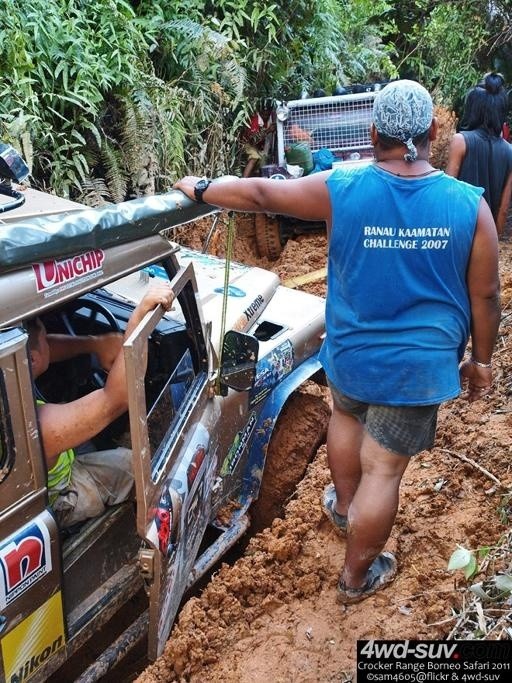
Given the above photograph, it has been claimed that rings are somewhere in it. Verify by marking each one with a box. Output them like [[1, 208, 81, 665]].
[[479, 387, 486, 391]]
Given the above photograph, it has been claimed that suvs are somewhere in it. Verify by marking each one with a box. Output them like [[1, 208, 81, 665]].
[[255, 82, 391, 260], [1, 144, 327, 682]]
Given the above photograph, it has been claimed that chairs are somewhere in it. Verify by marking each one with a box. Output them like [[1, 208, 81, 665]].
[[56, 499, 138, 616]]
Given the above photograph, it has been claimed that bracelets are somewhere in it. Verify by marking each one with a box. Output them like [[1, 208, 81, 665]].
[[470, 355, 493, 373]]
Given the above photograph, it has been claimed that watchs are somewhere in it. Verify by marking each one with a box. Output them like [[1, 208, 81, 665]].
[[192, 175, 214, 205]]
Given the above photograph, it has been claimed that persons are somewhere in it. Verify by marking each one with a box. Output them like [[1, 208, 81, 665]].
[[169, 77, 506, 614], [445, 70, 512, 239], [21, 279, 179, 533], [234, 79, 386, 178]]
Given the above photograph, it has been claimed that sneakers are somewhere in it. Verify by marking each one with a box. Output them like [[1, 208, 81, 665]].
[[337, 552, 398, 604], [321, 484, 348, 538]]
